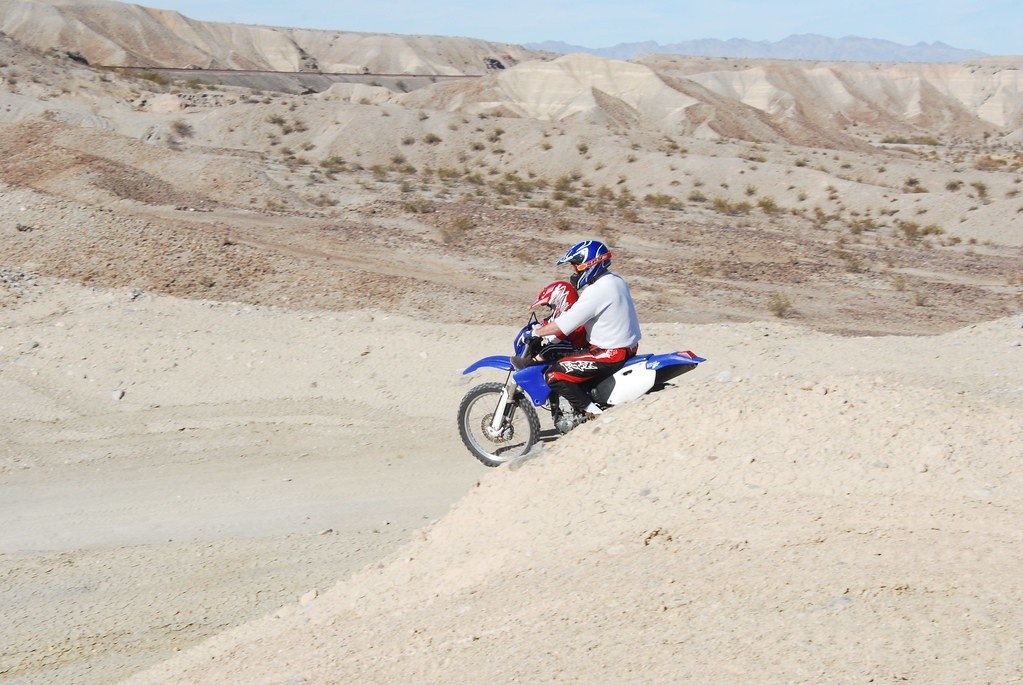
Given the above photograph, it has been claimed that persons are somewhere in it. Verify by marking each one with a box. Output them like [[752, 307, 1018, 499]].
[[510, 240, 643, 434]]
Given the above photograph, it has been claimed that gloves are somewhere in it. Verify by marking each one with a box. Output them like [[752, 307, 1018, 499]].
[[516, 329, 533, 347]]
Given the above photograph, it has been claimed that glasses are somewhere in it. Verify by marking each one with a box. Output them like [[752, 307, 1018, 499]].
[[571, 262, 580, 275]]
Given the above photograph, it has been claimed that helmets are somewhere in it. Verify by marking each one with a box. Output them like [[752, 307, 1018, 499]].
[[555, 240, 613, 291], [528, 280, 580, 328]]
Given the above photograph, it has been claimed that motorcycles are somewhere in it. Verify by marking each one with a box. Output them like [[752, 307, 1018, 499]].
[[457, 312, 706, 467]]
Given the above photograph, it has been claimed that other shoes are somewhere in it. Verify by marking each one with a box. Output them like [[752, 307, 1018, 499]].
[[509, 356, 543, 370]]
